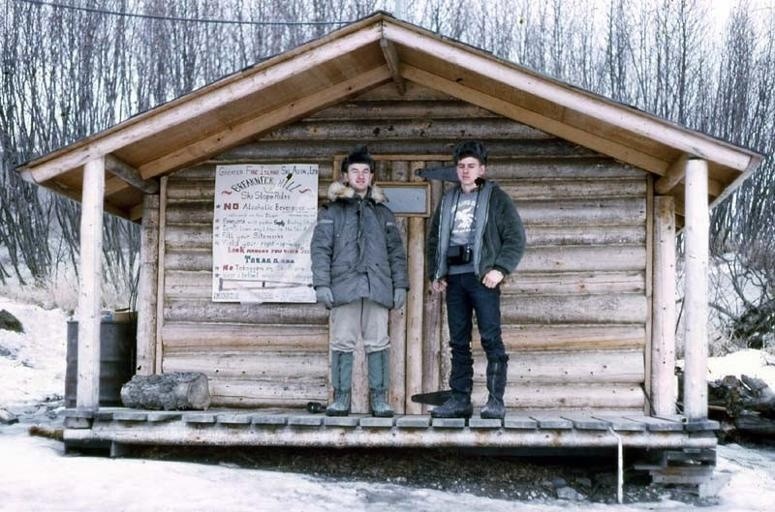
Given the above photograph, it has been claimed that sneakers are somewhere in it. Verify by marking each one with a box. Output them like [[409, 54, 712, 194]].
[[326, 390, 351, 416], [369, 390, 394, 417]]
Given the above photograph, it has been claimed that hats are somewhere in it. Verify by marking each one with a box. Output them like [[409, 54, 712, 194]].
[[453, 139, 488, 161], [341, 152, 376, 173]]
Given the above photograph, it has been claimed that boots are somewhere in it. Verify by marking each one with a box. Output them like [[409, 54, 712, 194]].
[[429, 357, 476, 419], [478, 360, 509, 418]]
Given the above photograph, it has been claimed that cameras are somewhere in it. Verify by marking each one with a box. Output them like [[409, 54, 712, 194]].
[[445, 246, 473, 266]]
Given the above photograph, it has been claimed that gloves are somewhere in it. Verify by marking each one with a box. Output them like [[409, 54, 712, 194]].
[[394, 287, 406, 310], [316, 286, 334, 309]]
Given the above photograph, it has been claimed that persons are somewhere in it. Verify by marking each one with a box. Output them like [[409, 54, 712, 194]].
[[310, 150, 409, 416], [432, 148, 526, 418]]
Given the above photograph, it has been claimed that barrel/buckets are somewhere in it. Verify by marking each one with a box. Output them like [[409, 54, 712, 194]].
[[65, 320, 134, 410]]
